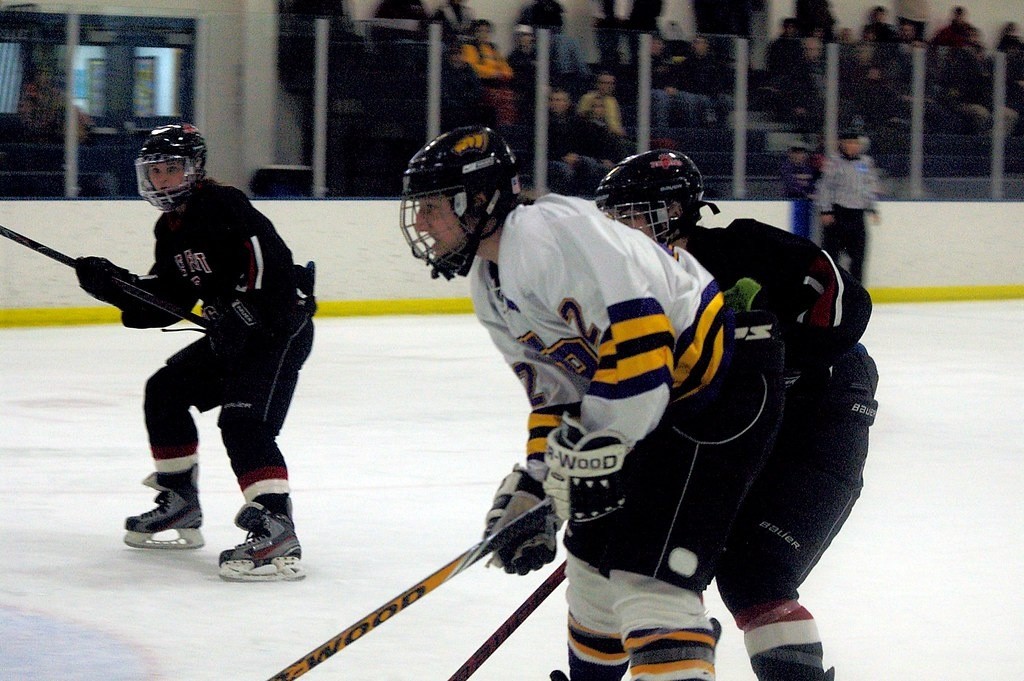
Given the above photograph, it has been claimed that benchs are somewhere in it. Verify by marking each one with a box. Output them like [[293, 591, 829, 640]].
[[503, 63, 1024, 202]]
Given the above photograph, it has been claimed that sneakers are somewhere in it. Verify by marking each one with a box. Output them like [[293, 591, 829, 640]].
[[217, 497, 307, 583], [812, 667, 835, 681], [710, 618, 721, 657], [549, 670, 570, 681], [123, 462, 206, 549]]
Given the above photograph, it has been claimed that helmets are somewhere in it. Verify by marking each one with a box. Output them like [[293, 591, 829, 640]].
[[595, 148, 705, 247], [133, 123, 208, 216], [400, 125, 520, 282]]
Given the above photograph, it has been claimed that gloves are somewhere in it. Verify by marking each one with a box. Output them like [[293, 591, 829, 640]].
[[76, 256, 139, 311], [542, 411, 636, 537], [481, 463, 564, 575], [719, 274, 780, 349], [205, 298, 274, 359]]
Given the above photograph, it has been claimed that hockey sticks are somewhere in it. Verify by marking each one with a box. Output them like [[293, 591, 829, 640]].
[[262, 500, 568, 681], [0, 225, 214, 329]]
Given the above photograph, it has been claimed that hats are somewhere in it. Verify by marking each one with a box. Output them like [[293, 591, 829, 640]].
[[510, 24, 534, 36]]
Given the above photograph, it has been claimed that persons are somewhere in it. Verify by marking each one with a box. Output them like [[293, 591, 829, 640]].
[[449, 4, 1023, 200], [74, 122, 319, 582], [398, 125, 785, 680], [815, 129, 880, 285], [592, 147, 881, 681]]
